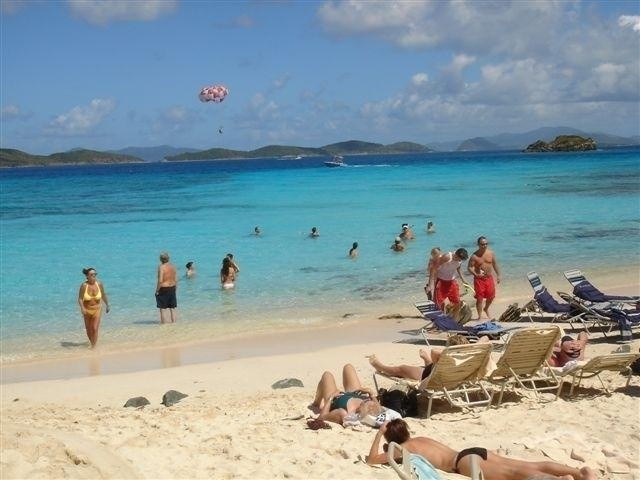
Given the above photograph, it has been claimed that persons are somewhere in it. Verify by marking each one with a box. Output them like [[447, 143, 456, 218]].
[[220, 258, 236, 290], [225, 253, 241, 273], [367, 418, 599, 480], [425, 221, 436, 232], [466, 235, 501, 320], [154, 252, 179, 325], [424, 248, 470, 309], [254, 224, 261, 235], [427, 247, 442, 269], [184, 260, 195, 278], [310, 363, 381, 424], [77, 266, 111, 349], [390, 237, 404, 252], [349, 240, 359, 257], [310, 225, 318, 236], [370, 334, 489, 381], [546, 329, 588, 367], [400, 222, 415, 240]]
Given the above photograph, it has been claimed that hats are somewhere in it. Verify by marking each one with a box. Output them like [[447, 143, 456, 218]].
[[562, 336, 580, 358]]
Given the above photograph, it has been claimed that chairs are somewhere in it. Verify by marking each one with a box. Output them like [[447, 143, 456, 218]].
[[539, 352, 636, 404], [373, 342, 493, 420], [387, 441, 483, 480], [412, 270, 640, 344], [472, 327, 561, 410]]
